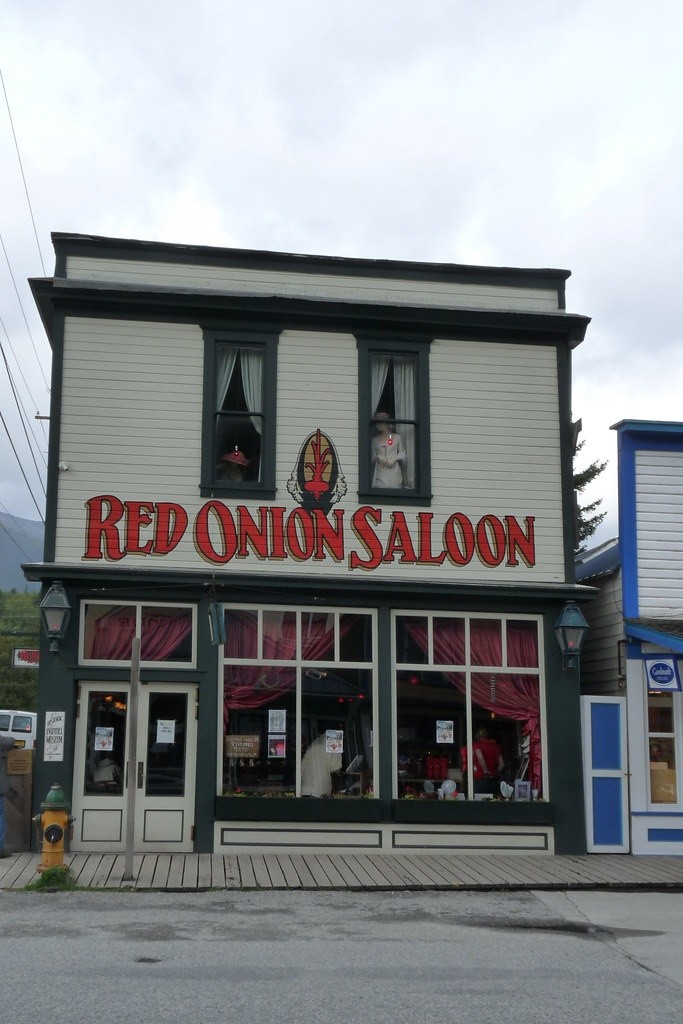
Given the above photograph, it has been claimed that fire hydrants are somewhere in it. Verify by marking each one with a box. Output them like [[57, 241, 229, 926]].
[[32, 784, 76, 870]]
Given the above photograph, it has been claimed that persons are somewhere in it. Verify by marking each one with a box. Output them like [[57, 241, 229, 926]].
[[216, 446, 248, 481], [303, 732, 342, 798], [474, 727, 504, 793], [90, 751, 122, 784], [0, 735, 14, 858], [370, 413, 406, 488], [460, 735, 467, 794]]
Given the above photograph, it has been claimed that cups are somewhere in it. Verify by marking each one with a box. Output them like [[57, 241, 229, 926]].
[[532, 789, 538, 801], [456, 793, 464, 800], [437, 789, 444, 800]]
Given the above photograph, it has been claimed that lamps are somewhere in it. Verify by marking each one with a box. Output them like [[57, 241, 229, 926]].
[[36, 580, 71, 652], [553, 599, 588, 669]]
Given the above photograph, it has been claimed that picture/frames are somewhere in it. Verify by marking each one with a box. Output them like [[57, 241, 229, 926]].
[[514, 781, 530, 801]]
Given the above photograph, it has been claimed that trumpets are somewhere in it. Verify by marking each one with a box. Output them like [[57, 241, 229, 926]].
[[306, 667, 327, 680]]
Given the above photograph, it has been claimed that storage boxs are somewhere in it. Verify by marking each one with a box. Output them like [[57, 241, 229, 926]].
[[649, 762, 675, 803]]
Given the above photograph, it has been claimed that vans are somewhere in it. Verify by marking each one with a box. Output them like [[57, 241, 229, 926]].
[[0, 709, 37, 750]]
